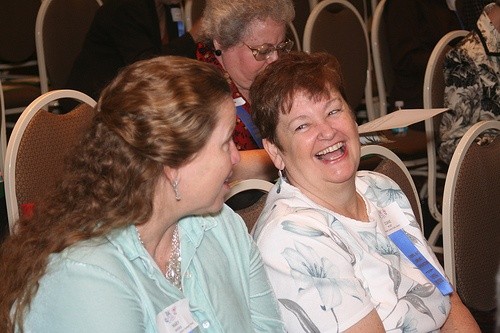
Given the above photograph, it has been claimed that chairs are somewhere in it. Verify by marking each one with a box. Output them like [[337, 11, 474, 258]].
[[0, 0, 500, 333]]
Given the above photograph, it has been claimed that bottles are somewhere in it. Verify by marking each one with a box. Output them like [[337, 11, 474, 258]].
[[392, 100, 408, 136]]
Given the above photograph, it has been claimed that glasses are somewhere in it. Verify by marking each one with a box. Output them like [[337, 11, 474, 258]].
[[234, 36, 294, 61]]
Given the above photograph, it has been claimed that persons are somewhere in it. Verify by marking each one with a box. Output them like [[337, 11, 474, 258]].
[[0, 56, 287, 333], [440, 0, 500, 164], [59, 0, 208, 114], [197, 0, 294, 209], [249, 53, 482, 333]]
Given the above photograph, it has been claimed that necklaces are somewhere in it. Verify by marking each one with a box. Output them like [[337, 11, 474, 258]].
[[136, 224, 181, 290]]
